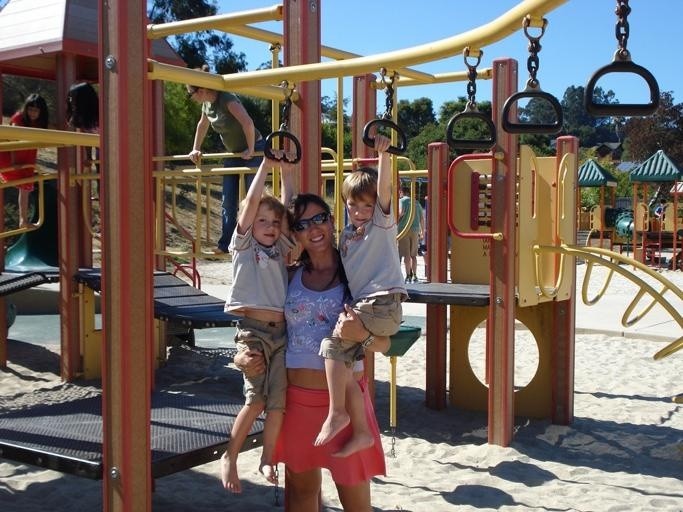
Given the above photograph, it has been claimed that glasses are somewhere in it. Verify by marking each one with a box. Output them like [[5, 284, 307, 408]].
[[187, 90, 198, 97], [293, 212, 331, 232]]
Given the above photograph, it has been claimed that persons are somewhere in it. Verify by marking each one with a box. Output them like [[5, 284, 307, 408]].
[[217, 149, 298, 492], [62, 81, 99, 138], [231, 192, 393, 511], [654, 198, 666, 221], [311, 131, 408, 459], [182, 64, 266, 258], [0, 91, 49, 230], [396, 183, 425, 284]]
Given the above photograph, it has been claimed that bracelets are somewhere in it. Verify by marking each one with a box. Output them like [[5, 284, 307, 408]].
[[360, 334, 375, 351]]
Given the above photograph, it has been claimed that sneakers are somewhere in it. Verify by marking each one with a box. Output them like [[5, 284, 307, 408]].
[[406, 274, 418, 284]]
